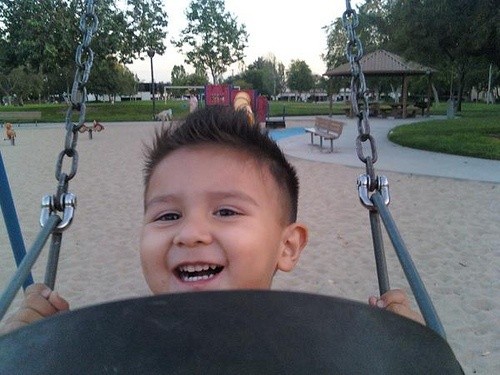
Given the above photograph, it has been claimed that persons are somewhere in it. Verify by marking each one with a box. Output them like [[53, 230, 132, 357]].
[[0, 104, 428, 341], [188, 89, 198, 114]]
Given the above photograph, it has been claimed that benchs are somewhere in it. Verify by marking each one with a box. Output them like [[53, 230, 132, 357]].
[[304, 116, 344, 154], [379, 107, 393, 118], [0, 111, 41, 128], [394, 109, 417, 119]]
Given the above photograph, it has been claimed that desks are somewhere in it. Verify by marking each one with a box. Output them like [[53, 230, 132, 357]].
[[389, 102, 410, 109]]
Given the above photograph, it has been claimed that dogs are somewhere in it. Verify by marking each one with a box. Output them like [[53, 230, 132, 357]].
[[155, 108, 173, 121], [5, 122, 16, 139]]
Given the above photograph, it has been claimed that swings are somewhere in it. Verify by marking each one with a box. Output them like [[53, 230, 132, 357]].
[[0, 2, 466, 375]]
